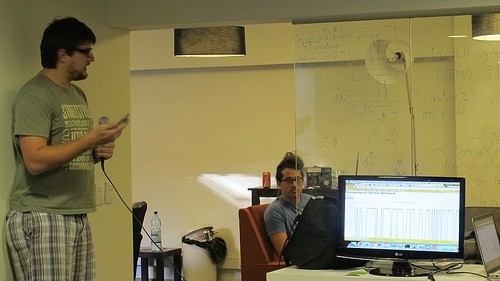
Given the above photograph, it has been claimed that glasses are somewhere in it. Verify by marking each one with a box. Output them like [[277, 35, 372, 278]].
[[60, 46, 92, 56], [278, 177, 303, 184]]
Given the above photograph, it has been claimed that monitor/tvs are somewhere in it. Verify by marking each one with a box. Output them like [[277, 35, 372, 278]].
[[336, 174, 466, 276]]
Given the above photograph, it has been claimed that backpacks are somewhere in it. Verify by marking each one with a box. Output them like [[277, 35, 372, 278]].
[[278, 195, 370, 270]]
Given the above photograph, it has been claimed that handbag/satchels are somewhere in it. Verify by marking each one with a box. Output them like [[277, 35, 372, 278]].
[[210, 237, 227, 264]]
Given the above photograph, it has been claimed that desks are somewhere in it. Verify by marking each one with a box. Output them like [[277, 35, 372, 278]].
[[266, 258, 500, 281], [248, 185, 338, 205], [138, 247, 182, 281]]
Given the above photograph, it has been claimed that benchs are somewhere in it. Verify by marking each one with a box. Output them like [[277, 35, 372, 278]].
[[239, 203, 288, 281]]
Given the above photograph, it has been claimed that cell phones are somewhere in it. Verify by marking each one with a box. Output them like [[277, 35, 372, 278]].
[[116, 113, 129, 123]]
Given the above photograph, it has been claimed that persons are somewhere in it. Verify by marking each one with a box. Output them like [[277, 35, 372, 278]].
[[262, 152, 315, 264], [3, 15, 130, 281]]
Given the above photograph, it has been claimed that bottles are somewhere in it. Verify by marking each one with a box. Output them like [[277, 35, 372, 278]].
[[150, 210, 162, 252]]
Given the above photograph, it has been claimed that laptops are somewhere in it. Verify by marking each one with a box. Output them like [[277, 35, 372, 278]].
[[471, 212, 500, 279]]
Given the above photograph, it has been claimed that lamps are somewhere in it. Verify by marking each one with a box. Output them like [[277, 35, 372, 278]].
[[472, 13, 500, 40], [174, 25, 247, 57]]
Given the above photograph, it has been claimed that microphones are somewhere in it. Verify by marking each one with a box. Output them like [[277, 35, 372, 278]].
[[99, 116, 110, 169]]
